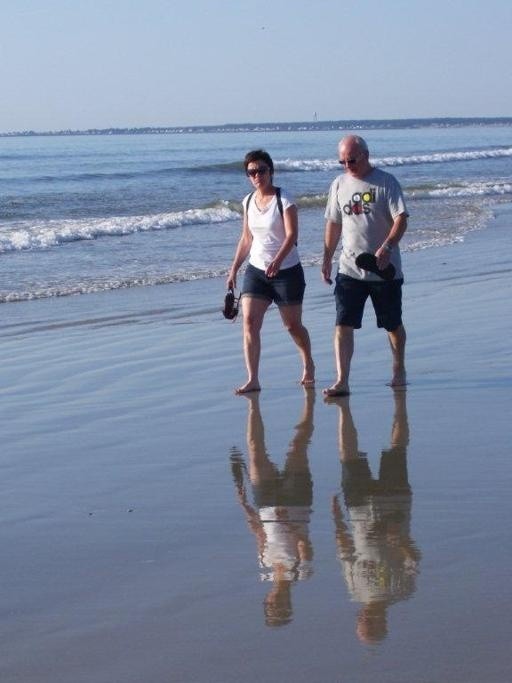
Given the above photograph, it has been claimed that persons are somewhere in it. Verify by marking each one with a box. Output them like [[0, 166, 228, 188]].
[[225, 151, 316, 394], [324, 392, 421, 646], [322, 135, 406, 397], [230, 384, 316, 628]]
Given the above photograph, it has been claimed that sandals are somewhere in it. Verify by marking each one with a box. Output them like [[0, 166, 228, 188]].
[[224, 289, 241, 322]]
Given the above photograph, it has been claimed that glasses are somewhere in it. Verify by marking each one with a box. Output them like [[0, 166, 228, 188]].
[[246, 167, 269, 177]]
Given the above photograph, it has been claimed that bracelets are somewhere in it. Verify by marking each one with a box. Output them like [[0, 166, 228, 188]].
[[381, 244, 395, 254]]
